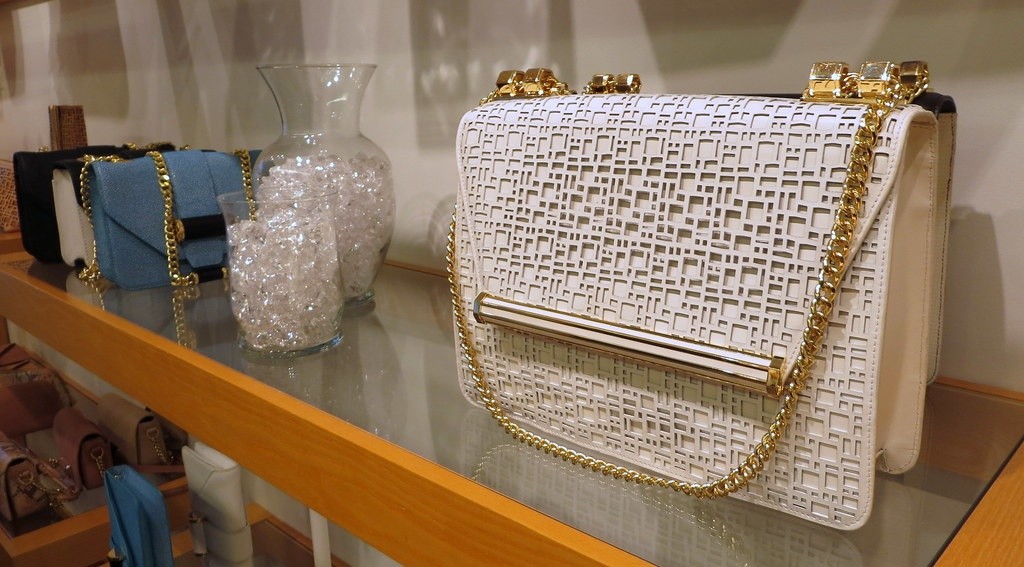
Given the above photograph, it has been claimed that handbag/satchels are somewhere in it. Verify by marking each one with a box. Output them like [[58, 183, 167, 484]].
[[45, 141, 177, 269], [0, 343, 29, 366], [12, 142, 137, 264], [0, 145, 49, 234], [446, 60, 958, 531], [0, 358, 46, 385], [0, 430, 80, 523], [52, 406, 115, 492], [96, 393, 169, 469], [0, 368, 74, 438], [87, 145, 278, 292]]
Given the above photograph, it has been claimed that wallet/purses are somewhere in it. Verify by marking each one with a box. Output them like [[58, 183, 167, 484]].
[[102, 464, 175, 567], [182, 440, 255, 567], [48, 104, 88, 150]]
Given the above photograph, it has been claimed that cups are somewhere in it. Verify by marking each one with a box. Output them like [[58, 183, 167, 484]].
[[216, 188, 344, 357]]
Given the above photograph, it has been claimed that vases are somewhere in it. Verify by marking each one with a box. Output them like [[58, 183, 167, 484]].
[[217, 186, 345, 359], [254, 65, 396, 314]]
